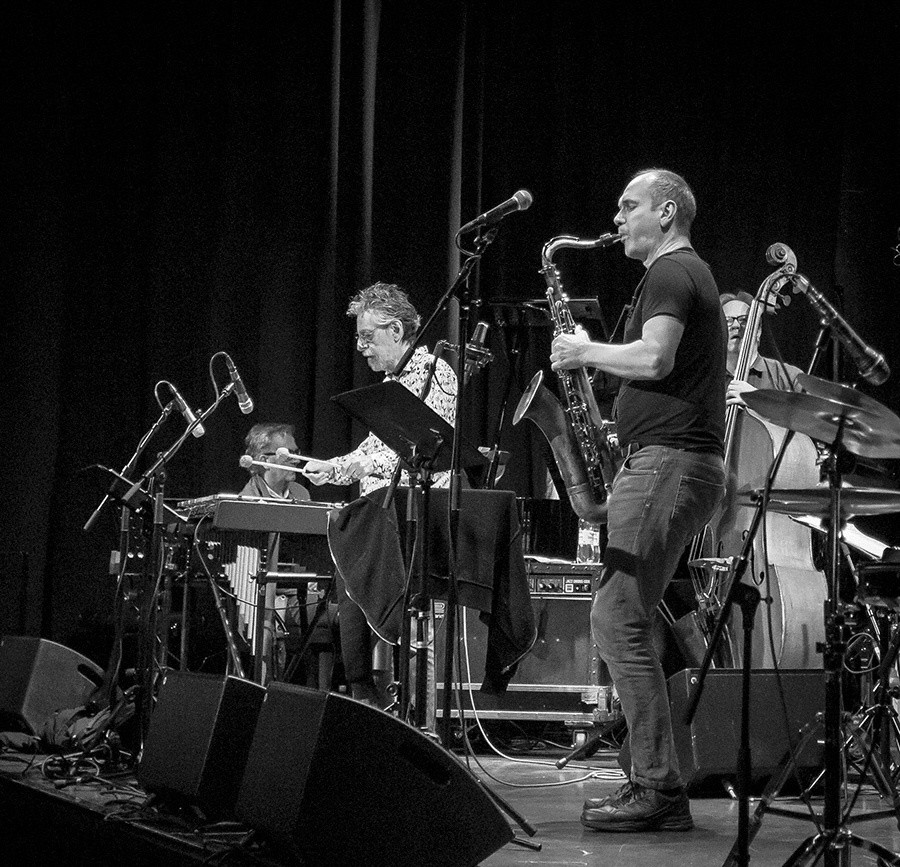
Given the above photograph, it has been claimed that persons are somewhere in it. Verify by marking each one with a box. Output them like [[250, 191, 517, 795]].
[[548, 166, 726, 834], [300, 283, 460, 710], [715, 290, 818, 407], [234, 422, 344, 645]]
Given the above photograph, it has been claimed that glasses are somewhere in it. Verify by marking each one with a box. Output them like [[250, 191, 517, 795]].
[[352, 325, 382, 341], [725, 314, 749, 327], [262, 447, 303, 460]]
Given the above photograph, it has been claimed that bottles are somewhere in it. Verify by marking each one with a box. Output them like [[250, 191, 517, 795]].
[[577, 518, 600, 564]]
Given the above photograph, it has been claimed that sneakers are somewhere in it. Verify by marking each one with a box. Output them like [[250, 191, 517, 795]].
[[579, 782, 694, 831], [585, 782, 635, 812]]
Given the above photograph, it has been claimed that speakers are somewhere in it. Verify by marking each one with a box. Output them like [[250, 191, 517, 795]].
[[0, 636, 105, 737], [614, 668, 825, 795], [136, 669, 267, 815], [230, 680, 515, 867]]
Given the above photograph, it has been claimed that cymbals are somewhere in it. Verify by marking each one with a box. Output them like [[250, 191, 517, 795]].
[[739, 478, 900, 514], [797, 372, 899, 429], [739, 388, 900, 457]]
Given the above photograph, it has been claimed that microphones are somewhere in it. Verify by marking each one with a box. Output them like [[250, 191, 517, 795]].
[[463, 321, 489, 389], [170, 384, 207, 438], [459, 189, 533, 239], [224, 355, 255, 414], [790, 276, 891, 387]]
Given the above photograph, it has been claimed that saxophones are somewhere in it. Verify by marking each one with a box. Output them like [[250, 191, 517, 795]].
[[511, 228, 622, 520]]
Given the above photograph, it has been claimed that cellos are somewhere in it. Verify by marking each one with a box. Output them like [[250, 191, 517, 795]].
[[672, 241, 830, 670]]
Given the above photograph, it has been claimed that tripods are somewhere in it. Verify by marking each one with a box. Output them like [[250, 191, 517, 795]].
[[35, 398, 179, 770], [782, 319, 898, 867], [55, 383, 236, 789], [390, 222, 506, 759], [283, 340, 465, 722]]
